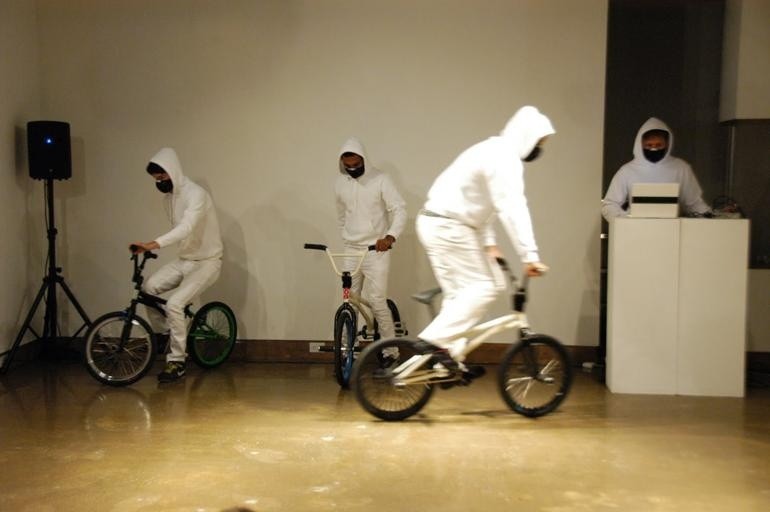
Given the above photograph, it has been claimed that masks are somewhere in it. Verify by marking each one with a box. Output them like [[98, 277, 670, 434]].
[[644, 149, 665, 163], [345, 165, 364, 178], [525, 147, 539, 161], [156, 179, 172, 193]]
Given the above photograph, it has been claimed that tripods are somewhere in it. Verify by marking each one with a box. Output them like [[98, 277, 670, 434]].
[[1, 180, 117, 379]]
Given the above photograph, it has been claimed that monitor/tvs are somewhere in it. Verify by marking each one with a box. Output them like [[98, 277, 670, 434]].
[[630, 183, 680, 217]]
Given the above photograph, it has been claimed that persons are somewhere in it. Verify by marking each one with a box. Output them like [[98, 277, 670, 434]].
[[127, 145, 224, 385], [598, 116, 715, 223], [415, 103, 557, 392], [331, 137, 407, 380]]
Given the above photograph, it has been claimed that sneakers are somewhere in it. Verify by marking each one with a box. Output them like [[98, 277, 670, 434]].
[[342, 347, 361, 368], [157, 335, 170, 354], [158, 361, 186, 383], [379, 357, 396, 368], [437, 366, 486, 389], [413, 341, 458, 372]]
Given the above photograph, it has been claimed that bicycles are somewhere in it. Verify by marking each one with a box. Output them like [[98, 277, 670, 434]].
[[345, 254, 570, 421], [301, 241, 402, 373], [75, 241, 241, 385]]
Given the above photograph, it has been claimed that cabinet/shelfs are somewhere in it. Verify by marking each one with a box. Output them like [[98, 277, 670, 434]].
[[604, 216, 751, 397], [719, 0, 769, 125]]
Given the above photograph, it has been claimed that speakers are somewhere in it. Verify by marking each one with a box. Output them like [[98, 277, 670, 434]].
[[27, 120, 73, 180]]
